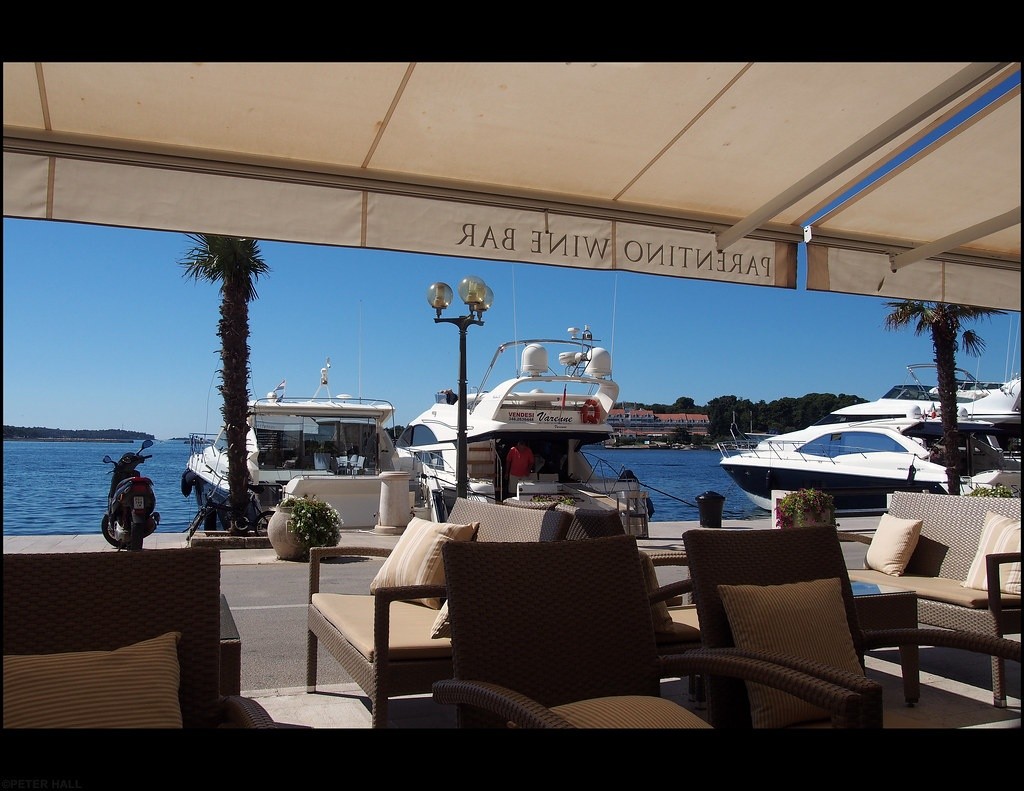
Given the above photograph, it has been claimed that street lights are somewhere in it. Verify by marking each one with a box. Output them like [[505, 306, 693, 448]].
[[428, 275, 495, 499]]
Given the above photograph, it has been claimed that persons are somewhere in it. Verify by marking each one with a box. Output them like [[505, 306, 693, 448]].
[[441, 389, 458, 405], [505, 440, 535, 497]]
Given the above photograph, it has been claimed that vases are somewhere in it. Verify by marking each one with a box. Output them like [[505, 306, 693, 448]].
[[267, 504, 312, 560], [793, 507, 831, 528]]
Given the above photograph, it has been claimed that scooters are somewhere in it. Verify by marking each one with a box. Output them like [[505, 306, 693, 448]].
[[101, 438, 161, 551]]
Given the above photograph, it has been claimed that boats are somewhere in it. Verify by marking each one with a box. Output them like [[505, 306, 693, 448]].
[[182, 356, 406, 535], [672, 442, 686, 450], [804, 362, 1022, 436], [394, 323, 655, 523], [710, 443, 739, 452], [715, 421, 1022, 519]]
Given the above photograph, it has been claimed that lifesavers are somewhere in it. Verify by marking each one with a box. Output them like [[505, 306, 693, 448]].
[[581, 398, 600, 424]]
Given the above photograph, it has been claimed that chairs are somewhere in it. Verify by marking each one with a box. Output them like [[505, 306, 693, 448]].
[[304, 491, 1023, 731], [336, 455, 365, 475], [0, 545, 277, 729], [282, 456, 298, 468]]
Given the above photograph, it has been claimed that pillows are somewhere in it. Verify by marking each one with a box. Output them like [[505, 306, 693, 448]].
[[506, 693, 714, 730], [716, 578, 866, 728], [370, 516, 480, 609], [861, 513, 923, 577], [2, 631, 183, 729], [961, 509, 1022, 596]]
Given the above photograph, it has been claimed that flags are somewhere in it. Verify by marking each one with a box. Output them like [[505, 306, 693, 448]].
[[273, 380, 285, 392], [932, 405, 936, 419]]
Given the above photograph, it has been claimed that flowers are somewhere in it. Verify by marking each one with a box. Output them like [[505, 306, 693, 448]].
[[773, 487, 841, 531], [276, 494, 344, 562], [964, 485, 1018, 499]]
